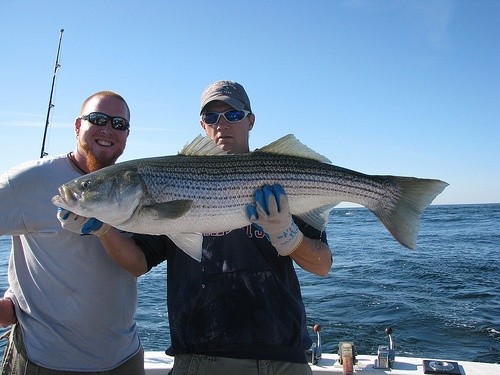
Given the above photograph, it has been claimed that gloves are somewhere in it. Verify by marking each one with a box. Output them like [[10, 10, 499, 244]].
[[246, 184, 304, 256], [57, 207, 111, 238]]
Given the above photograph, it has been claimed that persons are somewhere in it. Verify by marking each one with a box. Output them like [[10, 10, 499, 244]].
[[0, 91, 146, 375], [56, 80, 334, 375]]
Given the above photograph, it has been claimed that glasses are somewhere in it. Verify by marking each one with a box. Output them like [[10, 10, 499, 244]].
[[201, 108, 250, 125], [81, 112, 130, 132]]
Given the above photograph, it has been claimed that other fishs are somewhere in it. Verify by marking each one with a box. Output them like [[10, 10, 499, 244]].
[[51, 133, 450, 264]]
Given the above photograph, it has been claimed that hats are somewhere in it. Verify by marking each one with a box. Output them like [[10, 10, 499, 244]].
[[199, 80, 251, 115]]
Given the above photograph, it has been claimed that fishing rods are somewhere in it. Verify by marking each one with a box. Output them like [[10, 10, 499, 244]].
[[40, 29, 64, 158]]
